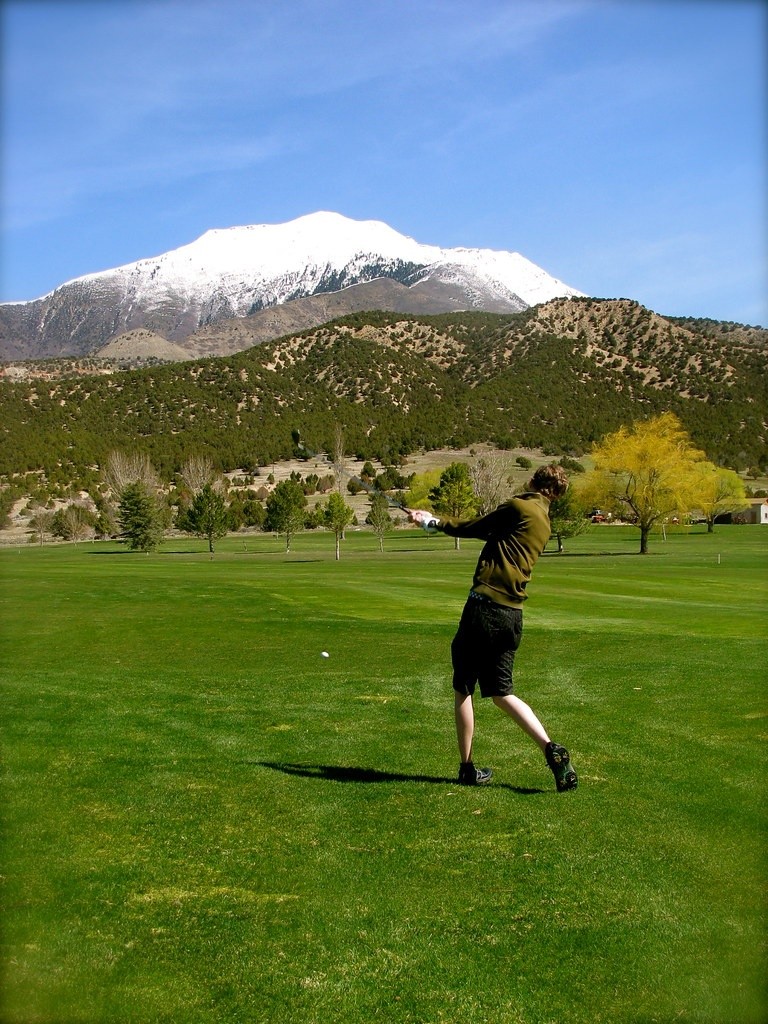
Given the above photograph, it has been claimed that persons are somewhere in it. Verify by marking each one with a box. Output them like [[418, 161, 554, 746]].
[[405, 464, 580, 792]]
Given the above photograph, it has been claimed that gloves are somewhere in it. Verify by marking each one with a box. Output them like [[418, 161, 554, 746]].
[[419, 510, 440, 535]]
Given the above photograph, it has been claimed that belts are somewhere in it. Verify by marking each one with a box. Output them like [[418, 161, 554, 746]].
[[467, 591, 491, 603]]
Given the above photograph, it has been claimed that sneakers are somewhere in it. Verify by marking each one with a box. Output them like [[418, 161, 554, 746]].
[[544, 744, 579, 792], [456, 766, 492, 785]]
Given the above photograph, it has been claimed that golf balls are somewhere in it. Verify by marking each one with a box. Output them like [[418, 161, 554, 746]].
[[322, 651, 330, 658]]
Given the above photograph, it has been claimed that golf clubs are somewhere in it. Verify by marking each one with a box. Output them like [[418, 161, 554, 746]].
[[291, 429, 436, 528]]
